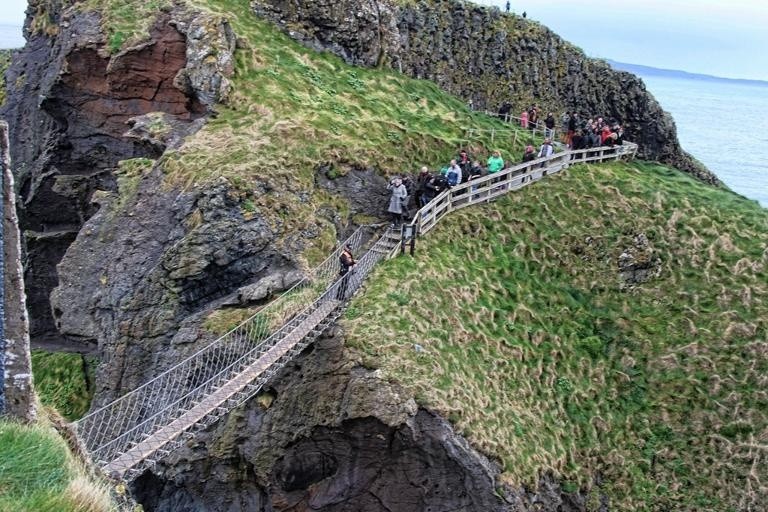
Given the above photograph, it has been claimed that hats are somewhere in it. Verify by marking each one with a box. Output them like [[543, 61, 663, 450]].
[[347, 243, 353, 250]]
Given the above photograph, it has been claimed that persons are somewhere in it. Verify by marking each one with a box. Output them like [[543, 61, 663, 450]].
[[498, 101, 626, 166], [335, 243, 358, 300], [384, 144, 510, 231], [504, 1, 510, 13], [521, 144, 537, 183], [538, 135, 553, 178]]
